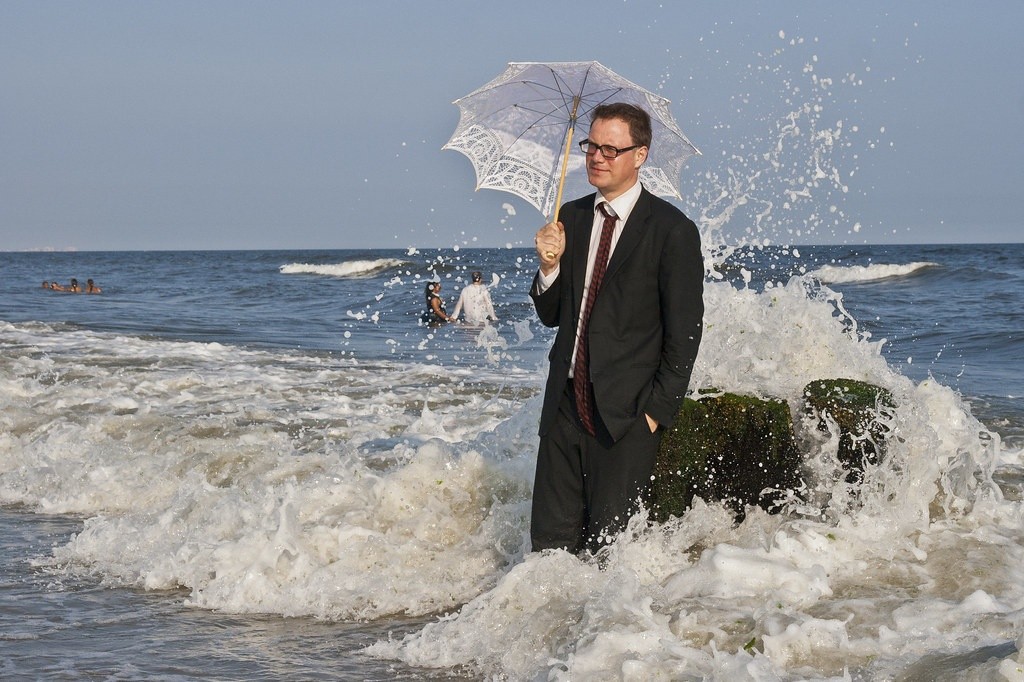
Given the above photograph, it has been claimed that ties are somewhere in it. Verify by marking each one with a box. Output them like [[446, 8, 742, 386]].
[[573, 202, 619, 437]]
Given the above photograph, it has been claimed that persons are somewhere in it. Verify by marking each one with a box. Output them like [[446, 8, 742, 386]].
[[421, 281, 449, 322], [449, 271, 498, 320], [527, 102, 704, 557], [68, 278, 81, 291], [43, 282, 64, 290], [86, 279, 101, 293]]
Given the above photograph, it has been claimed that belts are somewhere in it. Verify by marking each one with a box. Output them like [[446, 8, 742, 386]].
[[567, 378, 593, 394]]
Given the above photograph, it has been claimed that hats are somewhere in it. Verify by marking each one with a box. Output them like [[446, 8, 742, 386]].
[[472, 271, 482, 277]]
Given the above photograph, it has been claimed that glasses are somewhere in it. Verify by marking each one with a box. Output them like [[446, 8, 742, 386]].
[[579, 138, 642, 158]]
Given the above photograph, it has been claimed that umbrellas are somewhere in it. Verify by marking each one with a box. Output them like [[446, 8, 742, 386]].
[[441, 59, 702, 259]]
